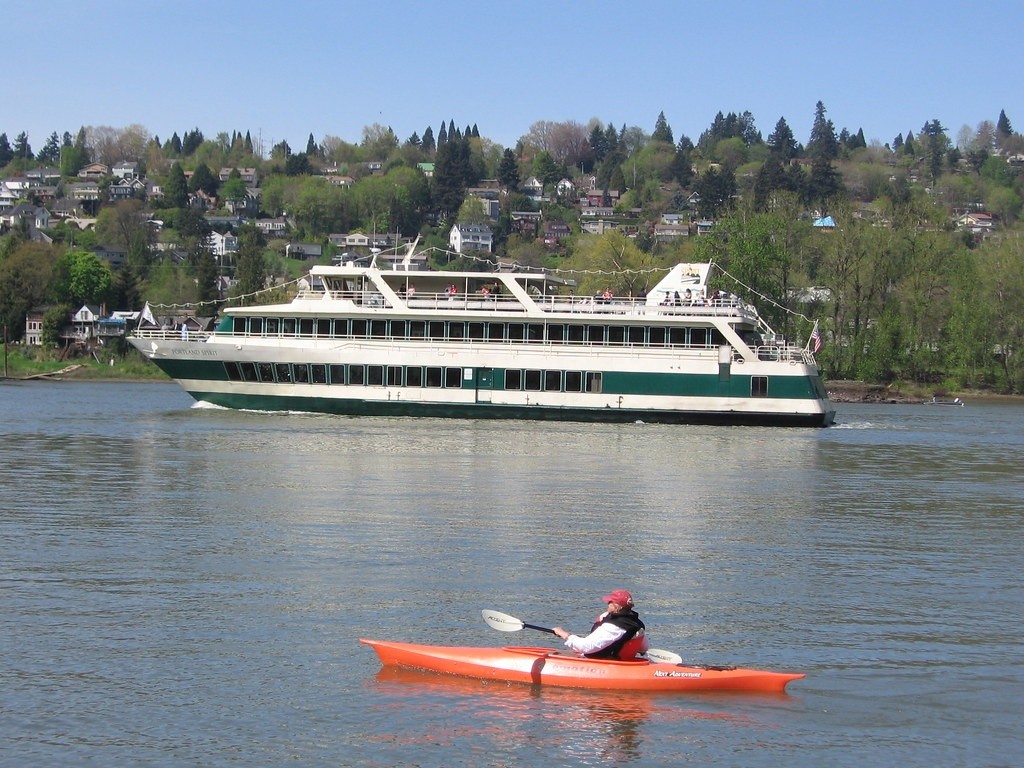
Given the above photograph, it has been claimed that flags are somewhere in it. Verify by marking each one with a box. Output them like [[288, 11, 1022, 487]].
[[142, 302, 156, 326], [811, 322, 821, 352]]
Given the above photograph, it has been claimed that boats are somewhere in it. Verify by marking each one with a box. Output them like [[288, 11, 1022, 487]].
[[359, 638, 806, 694]]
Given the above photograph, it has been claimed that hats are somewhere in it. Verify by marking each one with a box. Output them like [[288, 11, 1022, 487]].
[[602, 590, 633, 609]]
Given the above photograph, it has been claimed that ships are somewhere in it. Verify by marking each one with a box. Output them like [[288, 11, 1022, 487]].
[[126, 260, 836, 426]]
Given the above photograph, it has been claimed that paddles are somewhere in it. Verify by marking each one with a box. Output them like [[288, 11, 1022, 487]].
[[480, 608, 682, 666]]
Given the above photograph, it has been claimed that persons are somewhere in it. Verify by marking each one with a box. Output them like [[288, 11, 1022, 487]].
[[173, 320, 187, 341], [553, 590, 648, 662], [568, 287, 744, 317], [399, 282, 503, 301]]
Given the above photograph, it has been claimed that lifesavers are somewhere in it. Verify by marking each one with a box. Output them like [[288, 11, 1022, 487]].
[[788, 360, 797, 366], [737, 359, 744, 364]]
